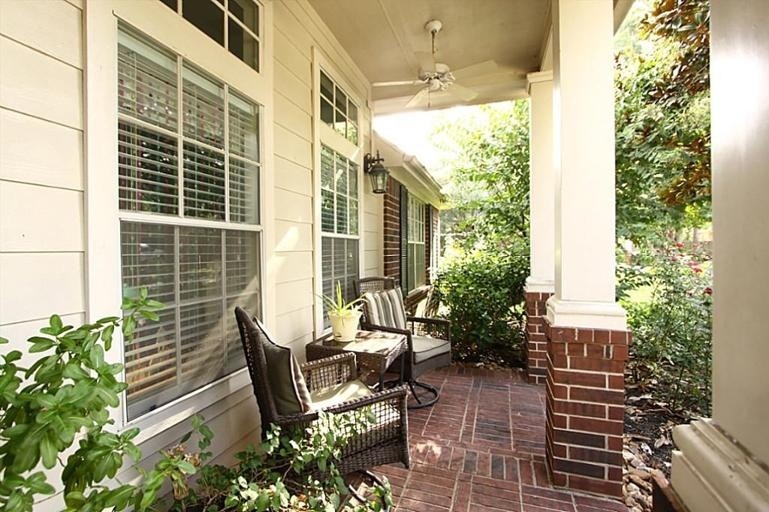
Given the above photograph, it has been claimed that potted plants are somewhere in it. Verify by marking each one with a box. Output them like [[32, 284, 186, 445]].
[[310, 281, 370, 342]]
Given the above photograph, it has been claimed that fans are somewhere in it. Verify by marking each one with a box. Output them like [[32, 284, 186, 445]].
[[371, 19, 495, 109]]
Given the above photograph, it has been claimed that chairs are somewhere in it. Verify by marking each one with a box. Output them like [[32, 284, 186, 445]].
[[234, 305, 412, 476], [353, 275, 452, 409]]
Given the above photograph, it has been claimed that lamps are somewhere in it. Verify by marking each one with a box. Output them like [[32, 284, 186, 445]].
[[426, 79, 441, 108], [364, 152, 391, 194]]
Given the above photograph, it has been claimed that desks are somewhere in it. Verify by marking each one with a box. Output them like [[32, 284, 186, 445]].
[[307, 330, 406, 395]]
